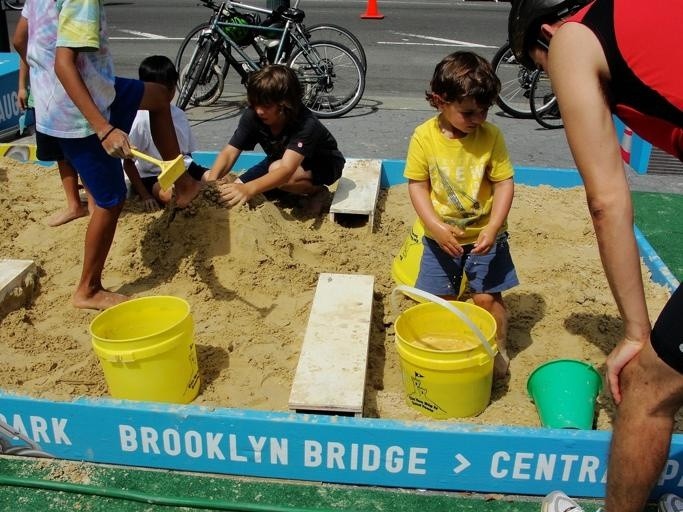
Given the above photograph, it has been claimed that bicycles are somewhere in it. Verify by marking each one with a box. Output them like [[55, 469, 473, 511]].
[[171, 0, 368, 116], [486, 28, 572, 129]]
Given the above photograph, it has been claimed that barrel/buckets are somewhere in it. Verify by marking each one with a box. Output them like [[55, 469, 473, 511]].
[[89, 296, 201, 405], [525, 359, 602, 430], [388, 286, 498, 419]]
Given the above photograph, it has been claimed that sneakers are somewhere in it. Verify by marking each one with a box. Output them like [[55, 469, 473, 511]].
[[541, 490, 606, 512], [659, 493, 683, 512]]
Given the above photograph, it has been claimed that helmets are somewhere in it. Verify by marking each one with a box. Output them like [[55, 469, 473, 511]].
[[243, 12, 261, 38], [222, 17, 248, 42], [261, 21, 282, 39], [508, 0, 593, 71]]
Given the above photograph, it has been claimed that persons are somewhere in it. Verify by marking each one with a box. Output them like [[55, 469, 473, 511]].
[[208, 64, 340, 219], [506, 0, 683, 511], [405, 49, 516, 383], [10, 0, 207, 311], [18, 60, 119, 231], [121, 54, 212, 215]]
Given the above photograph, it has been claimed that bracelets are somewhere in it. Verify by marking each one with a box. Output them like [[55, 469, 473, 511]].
[[97, 125, 117, 144]]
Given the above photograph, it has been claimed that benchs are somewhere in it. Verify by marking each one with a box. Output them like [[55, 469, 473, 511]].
[[330, 159, 382, 234], [287, 272, 375, 418], [0, 259, 36, 304]]
